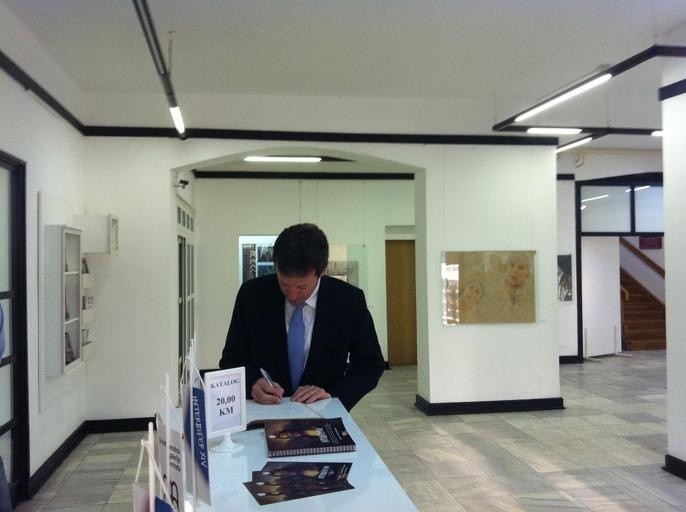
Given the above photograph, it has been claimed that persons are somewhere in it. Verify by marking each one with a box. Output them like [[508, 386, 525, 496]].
[[217, 221, 385, 415], [447, 252, 533, 323]]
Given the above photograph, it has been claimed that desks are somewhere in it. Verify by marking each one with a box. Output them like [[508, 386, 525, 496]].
[[174, 395, 419, 512]]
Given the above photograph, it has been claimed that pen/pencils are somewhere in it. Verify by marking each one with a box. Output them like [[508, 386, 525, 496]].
[[259, 367, 275, 390]]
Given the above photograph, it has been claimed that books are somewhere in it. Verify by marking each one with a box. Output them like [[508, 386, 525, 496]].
[[262, 415, 357, 458]]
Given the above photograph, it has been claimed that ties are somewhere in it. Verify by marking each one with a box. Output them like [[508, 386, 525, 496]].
[[288, 304, 304, 387]]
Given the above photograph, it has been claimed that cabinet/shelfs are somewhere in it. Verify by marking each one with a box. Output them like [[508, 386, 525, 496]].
[[43, 224, 94, 376]]
